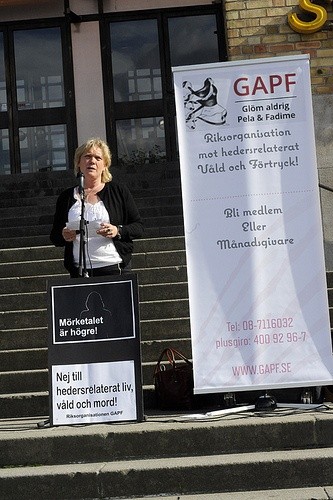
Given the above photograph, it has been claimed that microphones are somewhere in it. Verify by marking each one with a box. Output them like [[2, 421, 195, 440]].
[[77, 171, 85, 201]]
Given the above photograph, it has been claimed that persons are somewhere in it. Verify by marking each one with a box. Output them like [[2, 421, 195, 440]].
[[49, 138, 144, 279]]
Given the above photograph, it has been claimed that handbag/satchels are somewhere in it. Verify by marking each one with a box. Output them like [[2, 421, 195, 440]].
[[153, 347, 219, 412]]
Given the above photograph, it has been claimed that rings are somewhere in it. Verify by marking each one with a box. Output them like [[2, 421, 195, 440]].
[[107, 228, 110, 232]]
